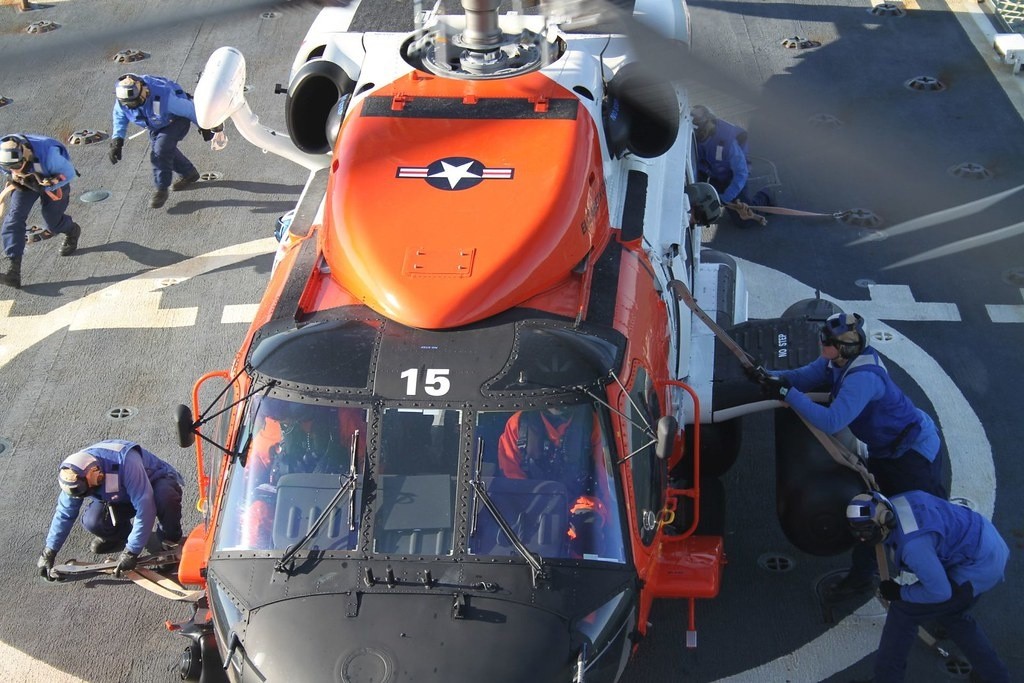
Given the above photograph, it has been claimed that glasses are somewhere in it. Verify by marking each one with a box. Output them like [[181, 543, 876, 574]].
[[852, 527, 883, 546], [817, 329, 842, 345]]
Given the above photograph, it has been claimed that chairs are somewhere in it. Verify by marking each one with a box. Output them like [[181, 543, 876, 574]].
[[475, 407, 598, 487], [301, 404, 407, 483]]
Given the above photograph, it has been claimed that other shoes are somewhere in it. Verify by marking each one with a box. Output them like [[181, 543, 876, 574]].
[[161, 538, 182, 551], [838, 566, 874, 598], [760, 185, 778, 220], [90, 523, 133, 554], [169, 168, 199, 190], [149, 186, 170, 210]]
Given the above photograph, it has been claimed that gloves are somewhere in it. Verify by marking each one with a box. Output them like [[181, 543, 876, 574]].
[[108, 138, 124, 165], [198, 128, 215, 142], [742, 362, 792, 401], [35, 552, 56, 582], [115, 553, 138, 578], [878, 577, 902, 601]]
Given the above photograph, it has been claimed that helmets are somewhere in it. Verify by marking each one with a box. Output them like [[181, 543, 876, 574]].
[[0, 134, 35, 173], [827, 312, 866, 344], [686, 182, 723, 226], [846, 492, 893, 548], [114, 73, 150, 110], [691, 105, 711, 132], [57, 452, 101, 499]]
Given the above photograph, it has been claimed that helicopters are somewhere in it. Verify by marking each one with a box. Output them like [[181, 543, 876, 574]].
[[0, 0, 1023, 681]]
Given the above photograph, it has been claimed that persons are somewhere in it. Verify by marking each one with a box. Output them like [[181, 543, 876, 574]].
[[107, 72, 215, 208], [757, 312, 940, 500], [0, 133, 82, 289], [691, 105, 780, 228], [497, 403, 612, 635], [243, 396, 369, 550], [845, 490, 1017, 683], [36, 439, 184, 583]]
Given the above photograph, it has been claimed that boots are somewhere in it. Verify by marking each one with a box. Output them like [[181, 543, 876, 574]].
[[0, 256, 21, 289], [58, 223, 80, 257]]
